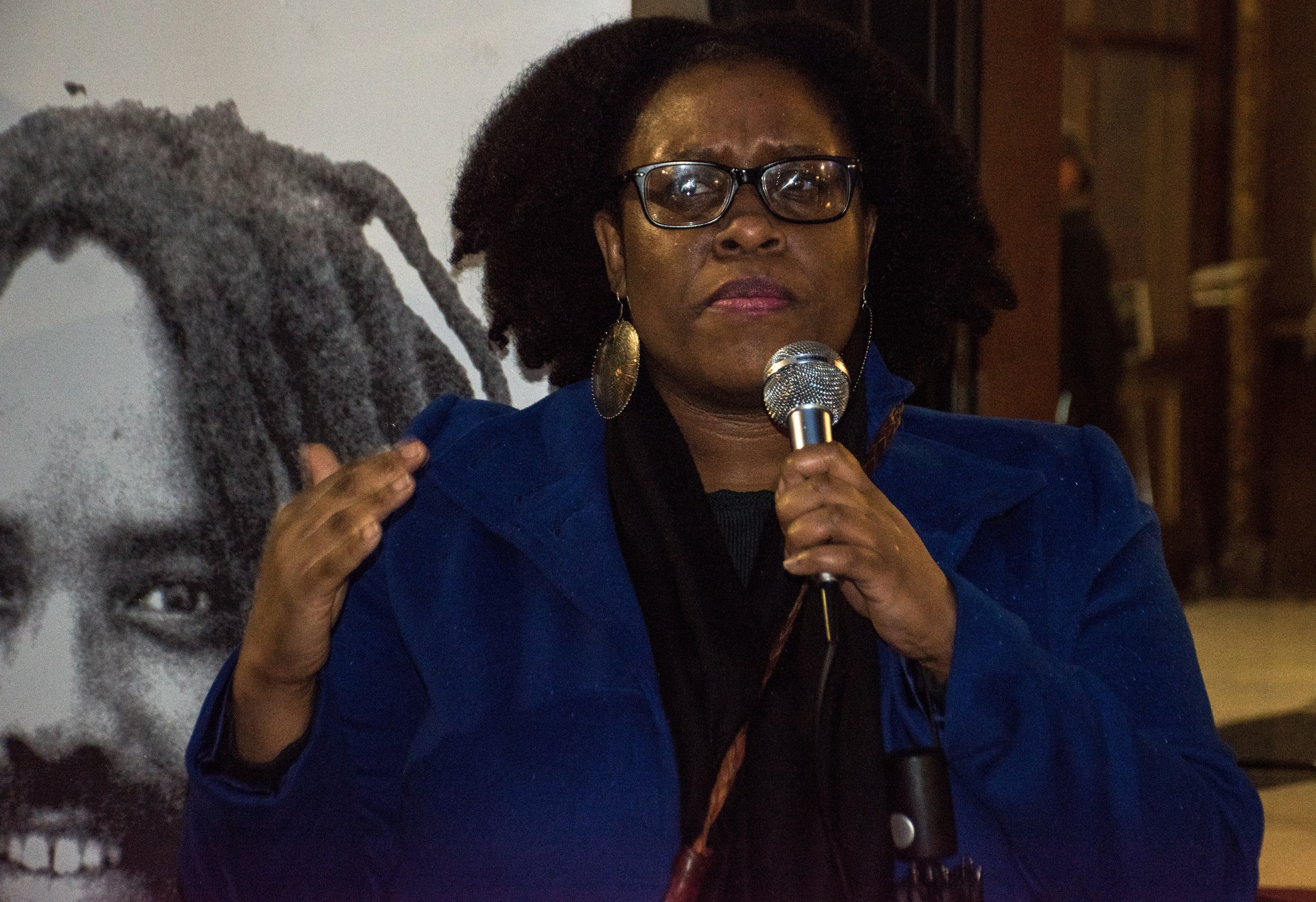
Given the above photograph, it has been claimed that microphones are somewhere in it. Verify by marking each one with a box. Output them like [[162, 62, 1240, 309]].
[[758, 338, 854, 646]]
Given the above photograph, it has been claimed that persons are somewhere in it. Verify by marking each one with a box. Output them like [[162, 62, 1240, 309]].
[[0, 96, 528, 902], [154, 7, 1266, 901]]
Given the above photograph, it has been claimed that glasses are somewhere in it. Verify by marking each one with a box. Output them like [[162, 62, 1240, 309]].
[[620, 154, 865, 230]]
